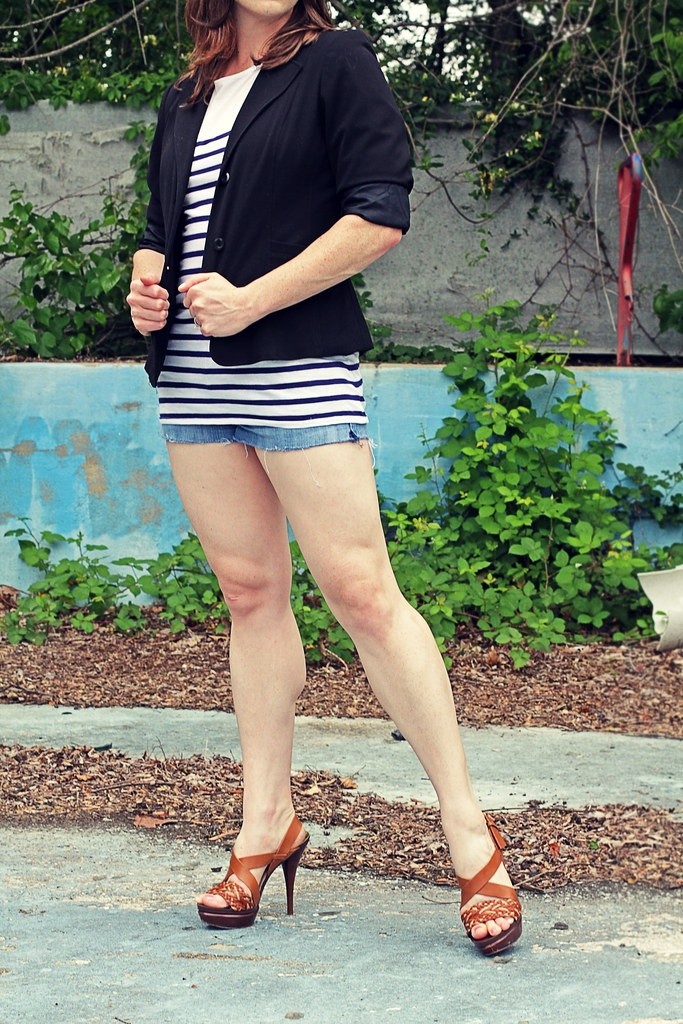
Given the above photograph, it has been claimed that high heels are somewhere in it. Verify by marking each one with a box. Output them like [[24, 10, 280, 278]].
[[196, 815, 310, 929], [454, 814, 522, 956]]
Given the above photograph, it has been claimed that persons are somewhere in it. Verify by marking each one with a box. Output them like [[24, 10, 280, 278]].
[[128, 0, 522, 957]]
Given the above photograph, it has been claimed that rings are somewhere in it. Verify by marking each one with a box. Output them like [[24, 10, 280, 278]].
[[194, 317, 201, 327]]
